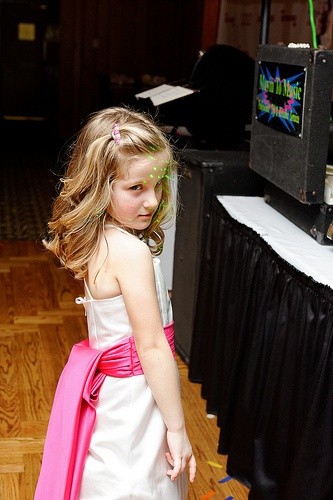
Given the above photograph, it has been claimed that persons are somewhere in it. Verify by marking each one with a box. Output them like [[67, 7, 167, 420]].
[[30, 107, 196, 500]]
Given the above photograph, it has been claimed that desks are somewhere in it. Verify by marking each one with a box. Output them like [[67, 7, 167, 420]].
[[169, 149, 253, 363], [188, 179, 332, 498]]
